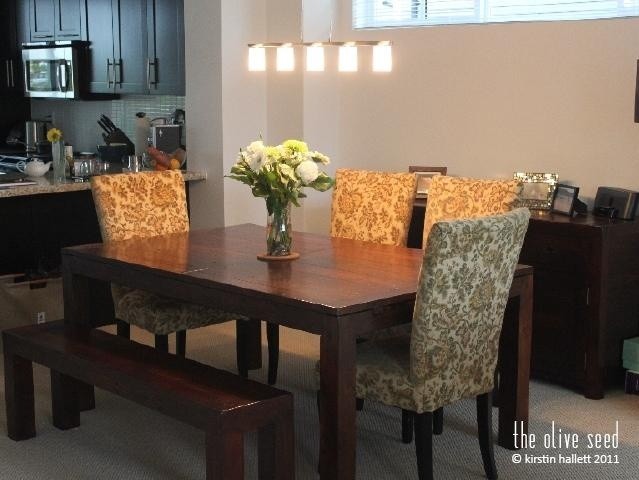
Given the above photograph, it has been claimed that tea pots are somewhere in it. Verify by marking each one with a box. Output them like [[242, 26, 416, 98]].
[[16, 156, 53, 176]]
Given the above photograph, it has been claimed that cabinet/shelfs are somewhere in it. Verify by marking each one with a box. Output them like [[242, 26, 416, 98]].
[[23, 0, 82, 41], [147, 1, 185, 96], [0, 0, 23, 98], [407, 196, 639, 401], [82, 0, 149, 95]]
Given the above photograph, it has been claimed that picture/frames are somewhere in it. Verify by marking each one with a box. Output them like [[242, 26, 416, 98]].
[[551, 183, 586, 218], [514, 171, 560, 211], [410, 165, 449, 200]]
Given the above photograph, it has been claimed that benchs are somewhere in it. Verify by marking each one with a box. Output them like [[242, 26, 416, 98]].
[[2, 320, 299, 479]]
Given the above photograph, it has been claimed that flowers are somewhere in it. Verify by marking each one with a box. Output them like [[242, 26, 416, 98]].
[[46, 127, 65, 144], [220, 134, 336, 200]]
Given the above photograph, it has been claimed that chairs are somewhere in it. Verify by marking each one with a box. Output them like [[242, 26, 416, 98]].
[[368, 177, 523, 444], [327, 167, 421, 250], [89, 171, 278, 383], [312, 202, 533, 479]]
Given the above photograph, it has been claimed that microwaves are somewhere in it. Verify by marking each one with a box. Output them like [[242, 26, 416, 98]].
[[20, 38, 85, 101]]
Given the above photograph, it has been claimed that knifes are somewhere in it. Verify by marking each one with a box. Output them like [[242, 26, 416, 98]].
[[96, 113, 117, 140]]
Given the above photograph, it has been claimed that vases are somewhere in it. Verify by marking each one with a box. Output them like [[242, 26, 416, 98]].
[[51, 142, 67, 186], [256, 193, 300, 261]]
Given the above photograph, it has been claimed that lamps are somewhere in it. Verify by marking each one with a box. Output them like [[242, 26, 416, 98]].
[[246, 0, 395, 76]]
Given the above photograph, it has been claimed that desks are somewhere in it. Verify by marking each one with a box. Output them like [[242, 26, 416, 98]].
[[59, 223, 536, 480]]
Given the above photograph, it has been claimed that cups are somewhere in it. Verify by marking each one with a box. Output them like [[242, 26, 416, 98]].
[[122, 152, 150, 172], [56, 158, 108, 182], [64, 146, 72, 159]]
